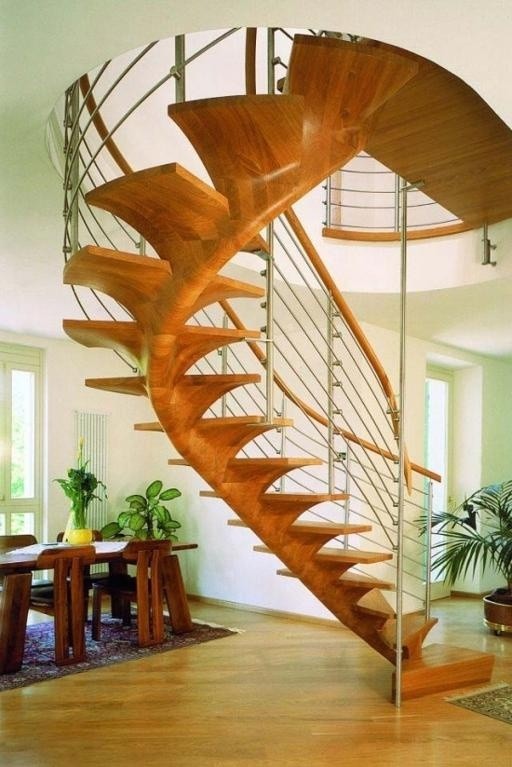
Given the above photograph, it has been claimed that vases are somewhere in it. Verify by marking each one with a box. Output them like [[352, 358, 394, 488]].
[[65, 504, 93, 545]]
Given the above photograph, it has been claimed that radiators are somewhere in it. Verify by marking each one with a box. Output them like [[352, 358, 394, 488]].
[[75, 410, 110, 573]]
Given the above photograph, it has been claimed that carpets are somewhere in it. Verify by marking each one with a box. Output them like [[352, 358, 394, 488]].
[[1, 604, 247, 694], [444, 680, 512, 724]]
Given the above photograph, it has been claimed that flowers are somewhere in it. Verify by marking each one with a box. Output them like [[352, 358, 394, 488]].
[[49, 437, 108, 528]]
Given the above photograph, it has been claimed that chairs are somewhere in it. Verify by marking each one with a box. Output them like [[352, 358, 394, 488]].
[[1, 529, 173, 666]]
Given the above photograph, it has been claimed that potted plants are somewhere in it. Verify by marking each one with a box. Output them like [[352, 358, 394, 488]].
[[411, 480, 511, 637]]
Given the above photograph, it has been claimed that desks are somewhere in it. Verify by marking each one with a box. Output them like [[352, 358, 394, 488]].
[[1, 540, 198, 677]]
